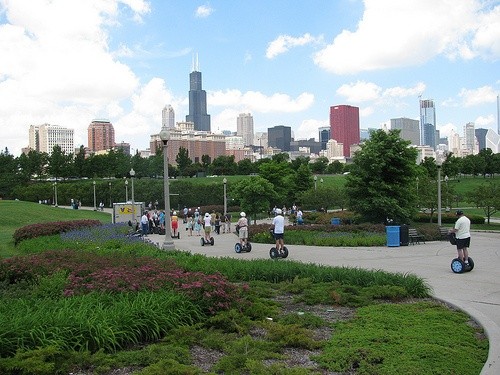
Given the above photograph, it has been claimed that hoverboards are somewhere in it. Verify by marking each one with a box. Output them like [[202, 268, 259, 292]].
[[171, 226, 180, 239], [235, 224, 252, 253], [200, 222, 215, 246], [449, 233, 475, 273], [268, 229, 288, 261]]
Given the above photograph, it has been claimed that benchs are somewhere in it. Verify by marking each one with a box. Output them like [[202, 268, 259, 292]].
[[438, 226, 454, 241], [409, 229, 425, 246]]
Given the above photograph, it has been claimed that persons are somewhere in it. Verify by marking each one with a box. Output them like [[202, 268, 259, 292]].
[[203, 213, 212, 243], [448, 210, 470, 263], [269, 208, 285, 255], [235, 212, 248, 249], [272, 202, 303, 222], [140, 200, 177, 237], [171, 211, 179, 237], [99, 201, 104, 210], [183, 205, 232, 236]]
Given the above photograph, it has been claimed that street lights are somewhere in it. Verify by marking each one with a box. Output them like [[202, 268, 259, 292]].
[[108, 181, 112, 208], [54, 182, 58, 208], [124, 179, 128, 203], [161, 123, 176, 252], [130, 166, 137, 229], [435, 155, 442, 227], [416, 177, 419, 210], [443, 174, 451, 212], [314, 174, 317, 192], [51, 183, 55, 206], [92, 180, 98, 212], [222, 178, 228, 217], [319, 177, 323, 189]]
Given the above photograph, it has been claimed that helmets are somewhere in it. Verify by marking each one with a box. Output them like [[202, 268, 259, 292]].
[[274, 208, 282, 214], [455, 210, 463, 215], [173, 212, 176, 215], [240, 212, 246, 216], [205, 213, 209, 217]]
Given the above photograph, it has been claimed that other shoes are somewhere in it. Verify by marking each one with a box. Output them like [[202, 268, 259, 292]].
[[208, 240, 211, 243], [241, 246, 243, 249], [281, 250, 284, 254], [278, 250, 280, 254], [465, 262, 469, 266], [204, 240, 207, 243], [244, 245, 247, 249]]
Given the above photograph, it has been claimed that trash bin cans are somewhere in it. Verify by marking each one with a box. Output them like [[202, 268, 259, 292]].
[[385, 226, 401, 247], [400, 224, 409, 246], [331, 218, 341, 225], [342, 218, 351, 225]]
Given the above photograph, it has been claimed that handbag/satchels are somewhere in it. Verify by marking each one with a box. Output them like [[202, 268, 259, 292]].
[[449, 232, 457, 245]]
